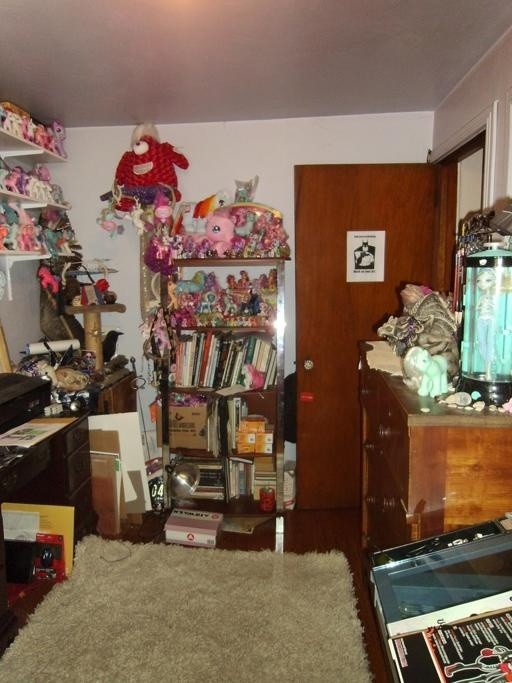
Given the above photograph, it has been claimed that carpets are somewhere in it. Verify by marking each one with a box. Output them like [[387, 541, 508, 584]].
[[0, 534, 374, 682]]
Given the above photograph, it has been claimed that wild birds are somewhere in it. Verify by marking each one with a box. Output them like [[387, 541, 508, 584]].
[[102, 329, 125, 362]]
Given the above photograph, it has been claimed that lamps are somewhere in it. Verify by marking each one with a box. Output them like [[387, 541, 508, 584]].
[[165, 459, 201, 499]]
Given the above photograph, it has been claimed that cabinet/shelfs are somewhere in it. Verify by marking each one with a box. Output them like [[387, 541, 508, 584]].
[[357, 339, 512, 579], [145, 255, 284, 524], [1, 127, 74, 301], [0, 368, 136, 657]]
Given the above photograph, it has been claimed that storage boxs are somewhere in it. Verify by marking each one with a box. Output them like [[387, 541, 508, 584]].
[[155, 405, 213, 449], [163, 507, 223, 548]]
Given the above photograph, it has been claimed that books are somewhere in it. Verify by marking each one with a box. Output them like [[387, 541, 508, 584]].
[[166, 398, 272, 456], [175, 332, 280, 390], [169, 457, 277, 503]]
